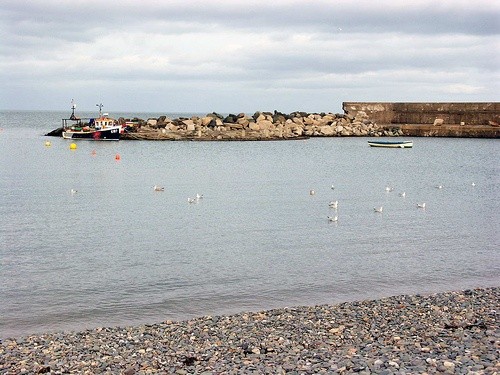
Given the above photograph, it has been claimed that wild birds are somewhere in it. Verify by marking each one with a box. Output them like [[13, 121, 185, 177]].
[[70, 183, 476, 223]]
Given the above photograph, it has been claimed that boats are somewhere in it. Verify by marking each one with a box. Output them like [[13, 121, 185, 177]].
[[367, 140, 414, 149], [62, 103, 128, 141]]
